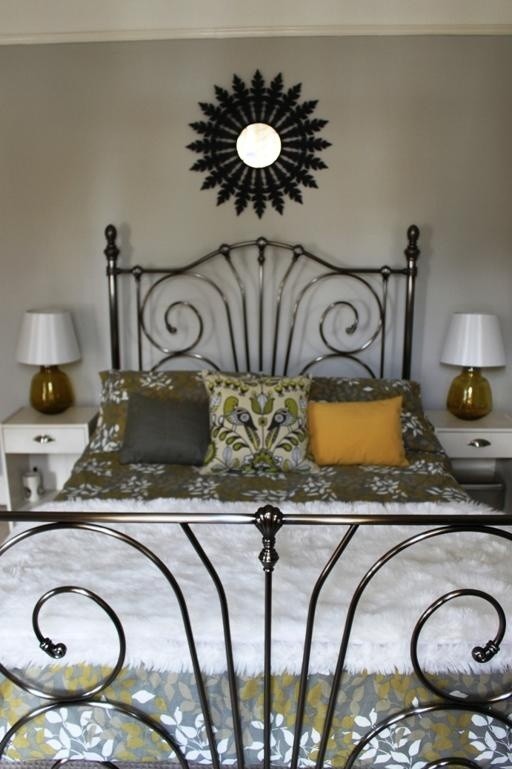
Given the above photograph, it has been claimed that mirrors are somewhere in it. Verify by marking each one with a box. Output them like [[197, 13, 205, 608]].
[[185, 66, 334, 222]]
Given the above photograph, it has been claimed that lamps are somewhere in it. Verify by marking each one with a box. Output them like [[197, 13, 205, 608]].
[[436, 312, 511, 423], [14, 305, 85, 415]]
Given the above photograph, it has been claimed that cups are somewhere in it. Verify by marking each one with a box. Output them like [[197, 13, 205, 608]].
[[21, 472, 44, 503]]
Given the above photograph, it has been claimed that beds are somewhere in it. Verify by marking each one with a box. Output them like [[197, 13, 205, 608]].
[[1, 225, 511, 769]]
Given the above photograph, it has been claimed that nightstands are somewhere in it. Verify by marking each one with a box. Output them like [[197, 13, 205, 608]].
[[419, 406, 511, 510], [1, 407, 98, 533]]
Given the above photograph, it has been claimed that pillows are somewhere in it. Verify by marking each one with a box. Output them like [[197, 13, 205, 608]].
[[117, 391, 210, 468], [200, 367, 319, 477], [312, 373, 443, 452], [94, 367, 259, 454], [306, 394, 410, 470]]
